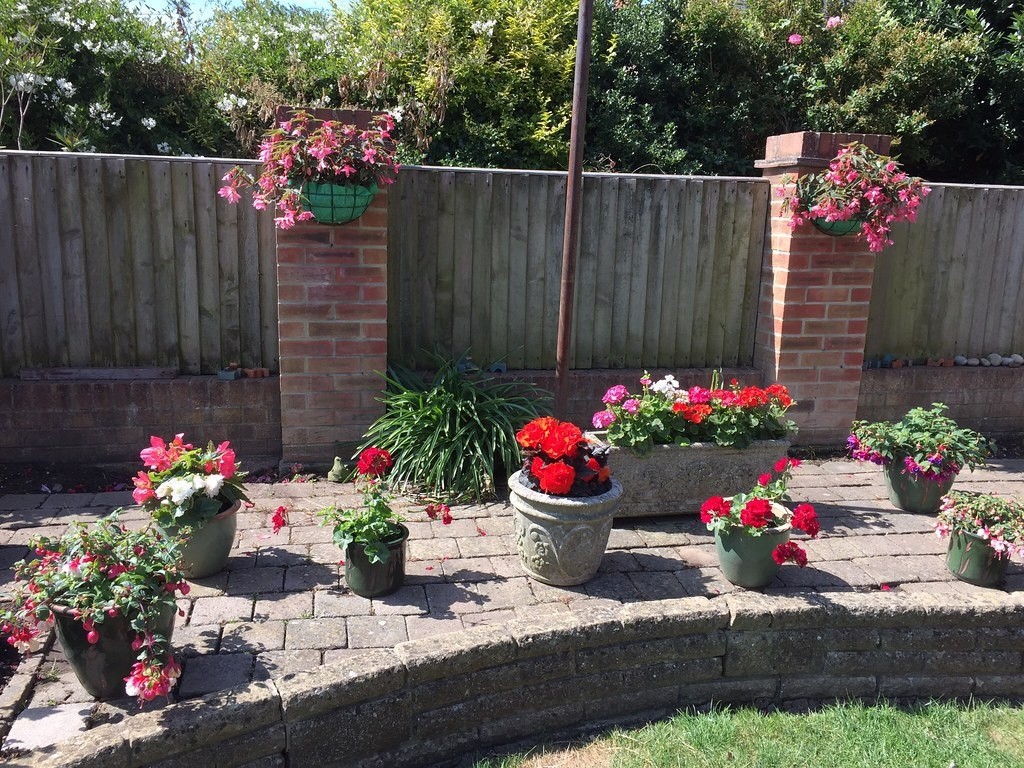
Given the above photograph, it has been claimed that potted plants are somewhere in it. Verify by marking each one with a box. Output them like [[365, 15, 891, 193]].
[[346, 347, 555, 507]]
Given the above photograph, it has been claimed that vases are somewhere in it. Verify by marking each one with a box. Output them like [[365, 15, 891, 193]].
[[504, 469, 626, 588], [714, 495, 795, 590], [290, 176, 375, 228], [583, 430, 794, 520], [150, 500, 238, 581], [803, 202, 872, 237], [946, 524, 1011, 596], [879, 448, 962, 517], [36, 599, 178, 702], [333, 522, 412, 600]]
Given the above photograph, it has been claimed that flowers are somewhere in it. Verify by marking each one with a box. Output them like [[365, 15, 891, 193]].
[[218, 108, 403, 231], [131, 433, 259, 527], [272, 447, 454, 566], [847, 403, 1006, 487], [934, 488, 1024, 562], [590, 368, 800, 459], [513, 414, 617, 499], [0, 507, 195, 706], [699, 458, 819, 571], [773, 141, 932, 253]]
